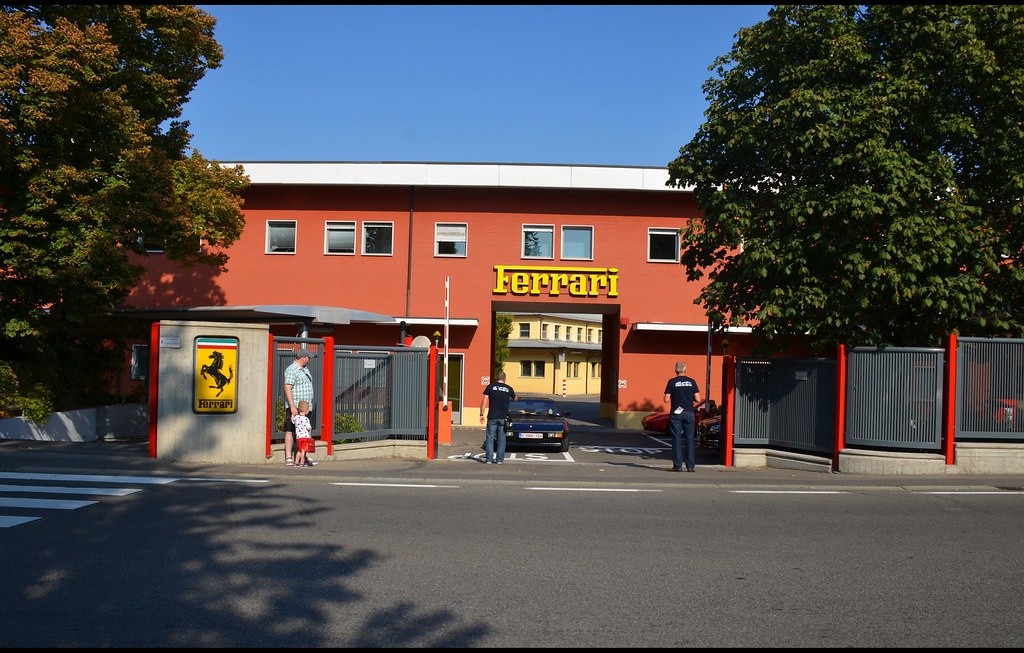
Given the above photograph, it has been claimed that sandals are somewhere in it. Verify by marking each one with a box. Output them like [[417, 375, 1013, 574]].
[[294, 462, 300, 467], [300, 464, 308, 467]]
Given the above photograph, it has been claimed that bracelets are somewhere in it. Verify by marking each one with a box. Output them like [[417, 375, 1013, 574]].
[[480, 414, 484, 416], [290, 403, 294, 406]]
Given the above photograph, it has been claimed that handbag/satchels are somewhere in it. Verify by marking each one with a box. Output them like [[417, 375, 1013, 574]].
[[505, 416, 510, 432]]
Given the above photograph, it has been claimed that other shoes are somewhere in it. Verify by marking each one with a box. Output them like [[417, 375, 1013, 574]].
[[304, 458, 319, 466], [497, 461, 504, 464], [687, 468, 695, 472], [671, 467, 682, 472], [285, 458, 294, 465], [485, 460, 491, 464]]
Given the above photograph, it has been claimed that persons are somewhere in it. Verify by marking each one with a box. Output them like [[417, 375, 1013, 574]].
[[479, 371, 518, 464], [284, 349, 319, 468], [663, 361, 700, 472]]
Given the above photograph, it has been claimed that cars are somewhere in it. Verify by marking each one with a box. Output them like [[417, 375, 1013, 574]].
[[641, 399, 717, 437], [498, 394, 572, 453], [696, 414, 722, 450]]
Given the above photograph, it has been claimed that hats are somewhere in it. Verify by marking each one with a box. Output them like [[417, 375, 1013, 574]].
[[296, 349, 315, 358]]
